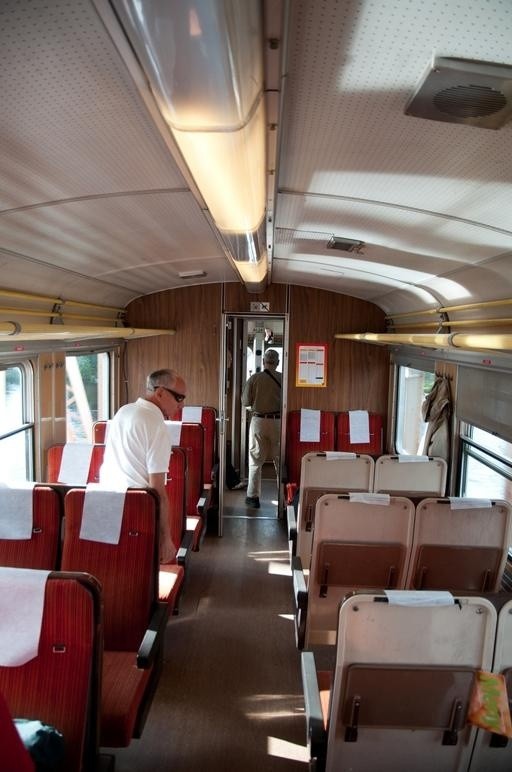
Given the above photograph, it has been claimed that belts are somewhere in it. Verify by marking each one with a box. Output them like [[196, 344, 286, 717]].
[[250, 413, 281, 420]]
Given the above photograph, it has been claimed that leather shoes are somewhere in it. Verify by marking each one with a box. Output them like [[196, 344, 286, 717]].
[[245, 495, 260, 508]]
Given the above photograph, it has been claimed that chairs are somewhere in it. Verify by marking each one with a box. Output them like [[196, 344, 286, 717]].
[[291, 494, 415, 650], [406, 498, 511, 599], [299, 588, 498, 769], [0, 684, 37, 770], [286, 410, 337, 502], [374, 456, 447, 498], [59, 489, 161, 748], [0, 406, 218, 611], [287, 451, 374, 569], [337, 410, 383, 456], [468, 601, 512, 769], [0, 565, 106, 771]]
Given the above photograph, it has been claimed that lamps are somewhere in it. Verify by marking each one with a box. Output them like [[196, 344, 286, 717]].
[[86, 1, 285, 296]]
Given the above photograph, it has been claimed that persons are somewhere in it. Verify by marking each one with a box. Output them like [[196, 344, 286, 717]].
[[239, 348, 283, 510], [92, 370, 189, 567]]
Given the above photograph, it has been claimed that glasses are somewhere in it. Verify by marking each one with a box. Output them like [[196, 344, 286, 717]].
[[154, 386, 186, 404]]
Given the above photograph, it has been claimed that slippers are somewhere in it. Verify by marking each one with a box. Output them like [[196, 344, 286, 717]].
[[233, 481, 247, 490]]
